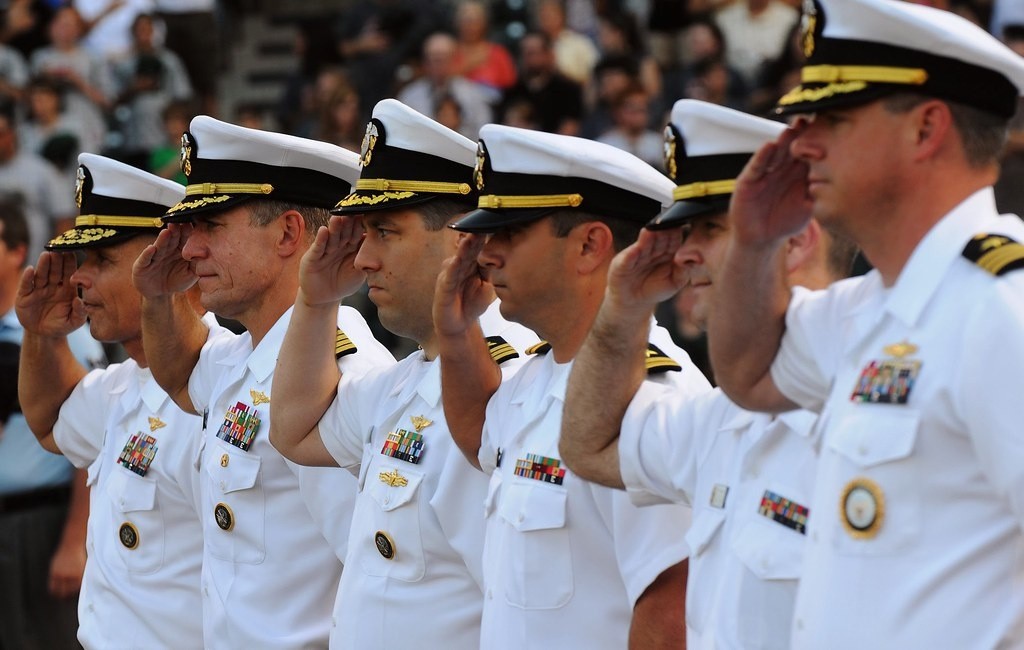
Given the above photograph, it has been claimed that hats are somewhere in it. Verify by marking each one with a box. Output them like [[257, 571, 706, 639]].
[[447, 124, 679, 233], [330, 100, 479, 215], [647, 99, 790, 230], [770, 0, 1024, 116], [44, 153, 186, 253], [160, 115, 361, 222]]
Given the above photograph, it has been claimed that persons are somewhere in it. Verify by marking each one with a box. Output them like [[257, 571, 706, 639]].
[[0, 0, 1024, 650]]
